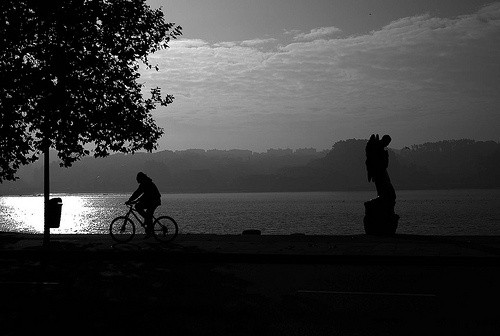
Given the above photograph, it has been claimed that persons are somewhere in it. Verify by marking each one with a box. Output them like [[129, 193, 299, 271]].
[[364, 134, 397, 212], [124, 172, 162, 237]]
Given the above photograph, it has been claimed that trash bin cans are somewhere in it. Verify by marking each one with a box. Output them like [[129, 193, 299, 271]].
[[49, 197, 63, 228]]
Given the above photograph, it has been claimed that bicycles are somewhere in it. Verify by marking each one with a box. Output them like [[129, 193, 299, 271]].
[[110, 201, 178, 244]]
